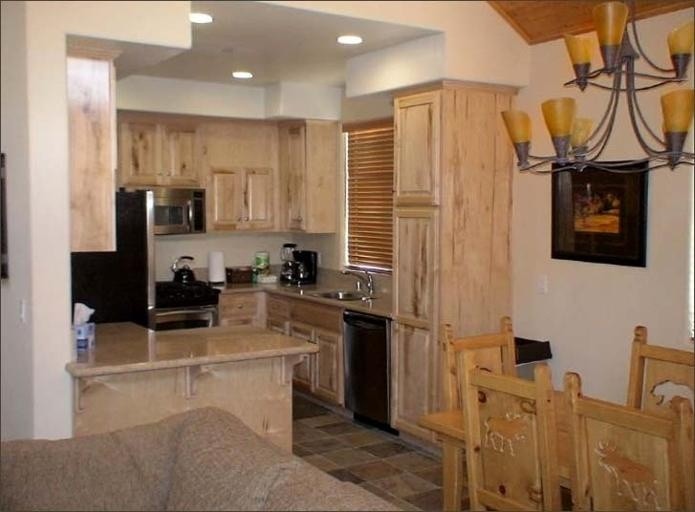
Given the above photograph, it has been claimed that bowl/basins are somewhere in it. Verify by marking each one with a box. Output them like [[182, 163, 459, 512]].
[[226, 267, 252, 284]]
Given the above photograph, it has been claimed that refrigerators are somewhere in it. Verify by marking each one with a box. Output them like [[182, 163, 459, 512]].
[[71, 190, 155, 332]]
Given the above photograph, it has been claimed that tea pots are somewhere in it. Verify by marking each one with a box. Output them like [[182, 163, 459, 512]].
[[171, 256, 197, 288]]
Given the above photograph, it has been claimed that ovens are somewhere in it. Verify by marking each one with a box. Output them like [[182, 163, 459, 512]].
[[152, 307, 219, 332]]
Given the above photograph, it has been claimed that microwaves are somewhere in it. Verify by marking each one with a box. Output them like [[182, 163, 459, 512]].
[[151, 186, 207, 235]]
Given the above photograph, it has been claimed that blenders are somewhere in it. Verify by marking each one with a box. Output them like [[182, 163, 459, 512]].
[[279, 243, 297, 281]]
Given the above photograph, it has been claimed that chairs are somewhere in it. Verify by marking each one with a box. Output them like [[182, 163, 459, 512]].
[[563, 372, 687, 510], [458, 348, 562, 512], [439, 314, 517, 412], [627, 325, 694, 510]]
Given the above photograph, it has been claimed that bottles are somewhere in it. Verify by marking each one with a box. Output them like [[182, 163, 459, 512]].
[[251, 268, 257, 283]]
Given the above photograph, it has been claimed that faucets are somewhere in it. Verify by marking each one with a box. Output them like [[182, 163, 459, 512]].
[[340, 264, 375, 294]]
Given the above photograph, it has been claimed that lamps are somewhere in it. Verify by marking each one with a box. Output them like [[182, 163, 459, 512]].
[[501, 1, 694, 174]]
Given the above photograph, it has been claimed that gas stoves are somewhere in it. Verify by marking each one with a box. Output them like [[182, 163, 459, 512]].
[[156, 281, 219, 306]]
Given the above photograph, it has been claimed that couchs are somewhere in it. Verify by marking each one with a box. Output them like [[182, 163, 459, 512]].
[[1, 405, 403, 511]]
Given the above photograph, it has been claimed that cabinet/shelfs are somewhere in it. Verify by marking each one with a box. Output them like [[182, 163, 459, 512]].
[[390, 80, 521, 464], [218, 288, 346, 406], [67, 44, 122, 254], [116, 112, 343, 236]]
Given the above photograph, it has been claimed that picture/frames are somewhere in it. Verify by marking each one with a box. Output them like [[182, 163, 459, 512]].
[[548, 157, 649, 268]]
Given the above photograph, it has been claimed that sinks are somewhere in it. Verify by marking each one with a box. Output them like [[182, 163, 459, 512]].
[[309, 290, 382, 301]]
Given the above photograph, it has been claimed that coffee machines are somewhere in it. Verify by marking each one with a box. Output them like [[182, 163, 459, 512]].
[[289, 249, 318, 285]]
